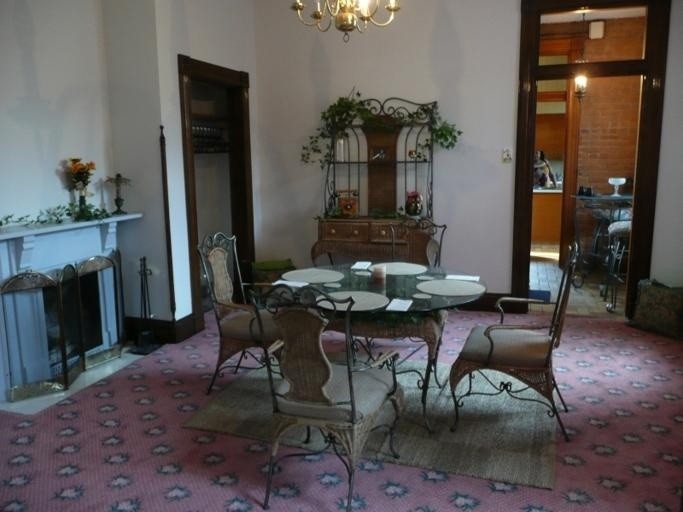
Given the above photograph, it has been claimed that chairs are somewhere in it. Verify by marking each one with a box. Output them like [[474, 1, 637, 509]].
[[438, 237, 580, 441], [193, 230, 300, 416], [240, 282, 403, 511], [566, 172, 653, 315], [346, 215, 449, 436]]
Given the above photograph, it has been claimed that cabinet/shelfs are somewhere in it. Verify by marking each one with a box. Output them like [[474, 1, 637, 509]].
[[306, 96, 448, 267], [0, 209, 147, 395]]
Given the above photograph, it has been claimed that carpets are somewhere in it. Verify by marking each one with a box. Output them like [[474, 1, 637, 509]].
[[175, 346, 564, 496], [0, 304, 680, 510]]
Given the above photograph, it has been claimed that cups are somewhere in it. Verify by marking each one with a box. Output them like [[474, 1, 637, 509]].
[[408, 149, 416, 158]]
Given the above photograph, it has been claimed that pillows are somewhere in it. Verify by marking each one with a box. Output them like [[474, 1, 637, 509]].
[[625, 276, 682, 341]]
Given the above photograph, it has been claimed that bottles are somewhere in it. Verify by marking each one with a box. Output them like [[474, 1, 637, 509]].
[[585, 187, 591, 196], [577, 185, 583, 196], [404, 191, 424, 217], [372, 264, 386, 283]]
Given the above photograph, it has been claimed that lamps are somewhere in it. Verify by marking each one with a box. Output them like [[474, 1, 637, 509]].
[[290, 0, 405, 46], [572, 73, 588, 102]]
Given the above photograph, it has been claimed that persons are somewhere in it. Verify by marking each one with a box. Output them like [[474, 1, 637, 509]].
[[533, 151, 558, 190]]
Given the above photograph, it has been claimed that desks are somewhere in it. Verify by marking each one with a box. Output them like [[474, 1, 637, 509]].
[[264, 254, 491, 440]]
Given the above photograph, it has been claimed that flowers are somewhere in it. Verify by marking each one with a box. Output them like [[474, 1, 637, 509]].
[[61, 156, 94, 195]]
